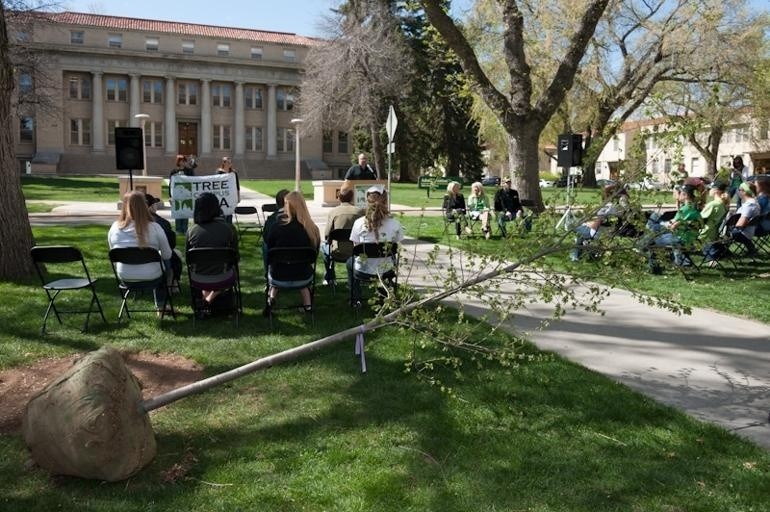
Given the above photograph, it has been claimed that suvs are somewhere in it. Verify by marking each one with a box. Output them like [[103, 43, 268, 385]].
[[558, 175, 582, 188]]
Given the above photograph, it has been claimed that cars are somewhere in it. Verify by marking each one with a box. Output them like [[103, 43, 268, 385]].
[[482, 175, 500, 186], [597, 179, 619, 188], [539, 178, 557, 188], [624, 177, 664, 192], [672, 174, 770, 192]]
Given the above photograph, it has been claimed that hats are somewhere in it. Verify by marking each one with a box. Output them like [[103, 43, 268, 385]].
[[708, 181, 727, 191], [145, 192, 161, 207], [676, 185, 697, 198]]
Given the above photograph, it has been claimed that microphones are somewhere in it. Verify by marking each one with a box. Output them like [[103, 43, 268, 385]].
[[366, 164, 374, 174]]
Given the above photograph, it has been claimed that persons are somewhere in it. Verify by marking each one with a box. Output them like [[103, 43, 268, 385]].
[[493, 179, 525, 240], [168, 156, 193, 233], [216, 157, 240, 223], [107, 186, 183, 317], [440, 180, 473, 241], [263, 189, 320, 318], [467, 180, 492, 240], [345, 153, 377, 180], [186, 193, 235, 315], [569, 156, 770, 278], [322, 184, 401, 309]]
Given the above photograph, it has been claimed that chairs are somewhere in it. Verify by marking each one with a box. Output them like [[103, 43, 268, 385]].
[[255, 204, 279, 247], [30, 245, 110, 335], [325, 228, 354, 296], [105, 247, 179, 325], [352, 242, 400, 306], [468, 206, 493, 238], [186, 249, 248, 329], [580, 198, 770, 285], [260, 246, 319, 323], [441, 203, 470, 239], [232, 206, 263, 249], [494, 209, 523, 237]]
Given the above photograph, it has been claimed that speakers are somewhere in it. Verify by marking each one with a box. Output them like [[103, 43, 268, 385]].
[[557, 133, 583, 167], [115, 127, 144, 170]]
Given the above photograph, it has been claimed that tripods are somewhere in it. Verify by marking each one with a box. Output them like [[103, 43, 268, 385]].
[[556, 168, 579, 231]]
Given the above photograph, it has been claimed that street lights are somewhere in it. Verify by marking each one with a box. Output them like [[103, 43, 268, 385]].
[[290, 118, 304, 193], [133, 114, 149, 176]]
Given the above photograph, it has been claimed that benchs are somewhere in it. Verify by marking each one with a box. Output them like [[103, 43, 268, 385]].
[[419, 176, 464, 199]]
